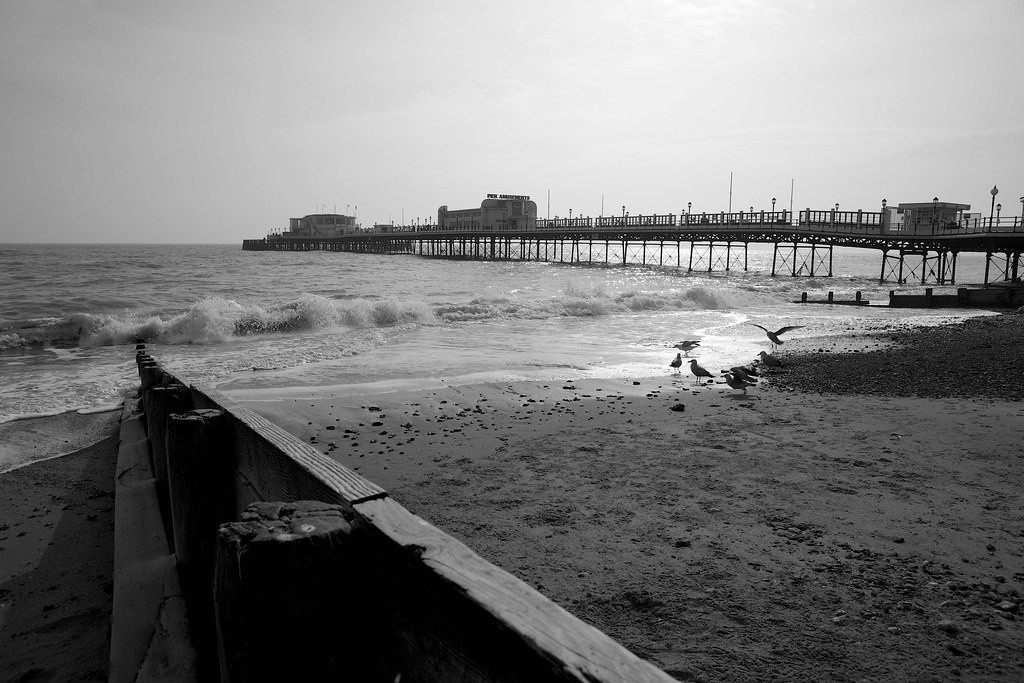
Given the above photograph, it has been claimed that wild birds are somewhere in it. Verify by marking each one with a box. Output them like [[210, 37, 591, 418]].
[[687, 359, 716, 383], [673, 340, 701, 356], [749, 323, 806, 345], [720, 367, 758, 395], [757, 351, 783, 372], [669, 353, 682, 374]]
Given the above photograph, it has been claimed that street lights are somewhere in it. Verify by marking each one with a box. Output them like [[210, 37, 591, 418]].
[[996, 203, 1002, 232], [682, 209, 685, 215], [622, 205, 626, 218], [688, 202, 692, 215], [771, 198, 776, 229], [835, 203, 840, 233], [880, 199, 887, 233], [569, 208, 573, 223], [749, 207, 754, 224], [931, 196, 938, 236], [1020, 196, 1024, 226], [988, 185, 998, 233]]
